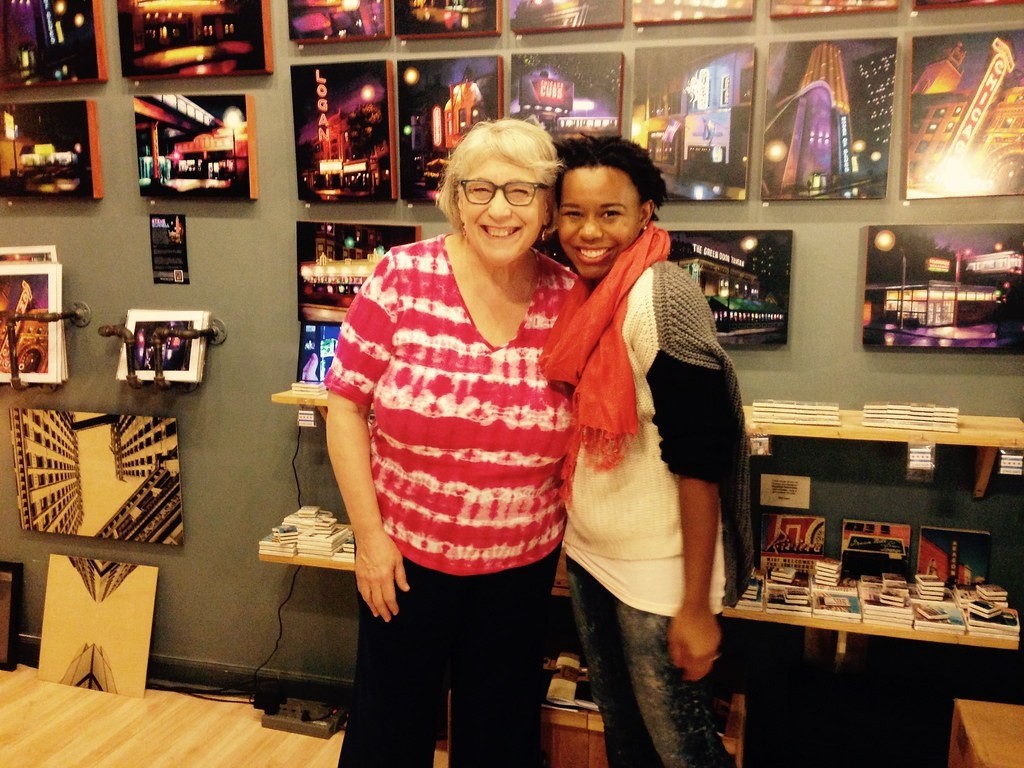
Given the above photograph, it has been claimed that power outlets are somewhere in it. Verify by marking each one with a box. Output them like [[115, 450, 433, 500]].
[[907, 442, 936, 481], [297, 405, 321, 428], [999, 449, 1024, 476], [746, 434, 772, 457]]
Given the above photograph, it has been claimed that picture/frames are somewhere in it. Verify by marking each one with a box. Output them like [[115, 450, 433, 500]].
[[760, 36, 898, 202], [396, 54, 504, 204], [393, 0, 503, 40], [116, 0, 274, 80], [508, 0, 624, 35], [0, 0, 109, 91], [38, 553, 159, 698], [631, 42, 756, 201], [290, 59, 399, 204], [0, 98, 105, 201], [8, 406, 185, 548], [296, 321, 343, 391], [0, 560, 24, 672], [296, 219, 422, 324], [509, 52, 624, 144], [770, 0, 901, 18], [905, 23, 1024, 201], [133, 93, 259, 202], [115, 308, 211, 384], [631, 0, 754, 25], [287, 0, 391, 43], [911, 0, 1024, 11]]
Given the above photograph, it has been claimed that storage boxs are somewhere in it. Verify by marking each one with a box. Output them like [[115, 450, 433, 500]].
[[447, 650, 588, 768], [588, 692, 746, 768]]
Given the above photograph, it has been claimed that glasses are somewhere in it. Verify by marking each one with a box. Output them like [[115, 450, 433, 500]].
[[458, 176, 547, 206]]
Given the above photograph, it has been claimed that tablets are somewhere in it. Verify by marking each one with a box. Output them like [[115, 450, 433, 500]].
[[297, 321, 342, 391]]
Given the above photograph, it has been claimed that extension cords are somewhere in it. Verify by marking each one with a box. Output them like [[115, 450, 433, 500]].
[[263, 698, 348, 739]]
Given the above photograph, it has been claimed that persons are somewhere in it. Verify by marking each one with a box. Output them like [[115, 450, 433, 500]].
[[323, 116, 591, 768], [549, 132, 763, 768]]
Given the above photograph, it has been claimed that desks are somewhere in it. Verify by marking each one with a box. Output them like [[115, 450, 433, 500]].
[[948, 699, 1024, 768]]
